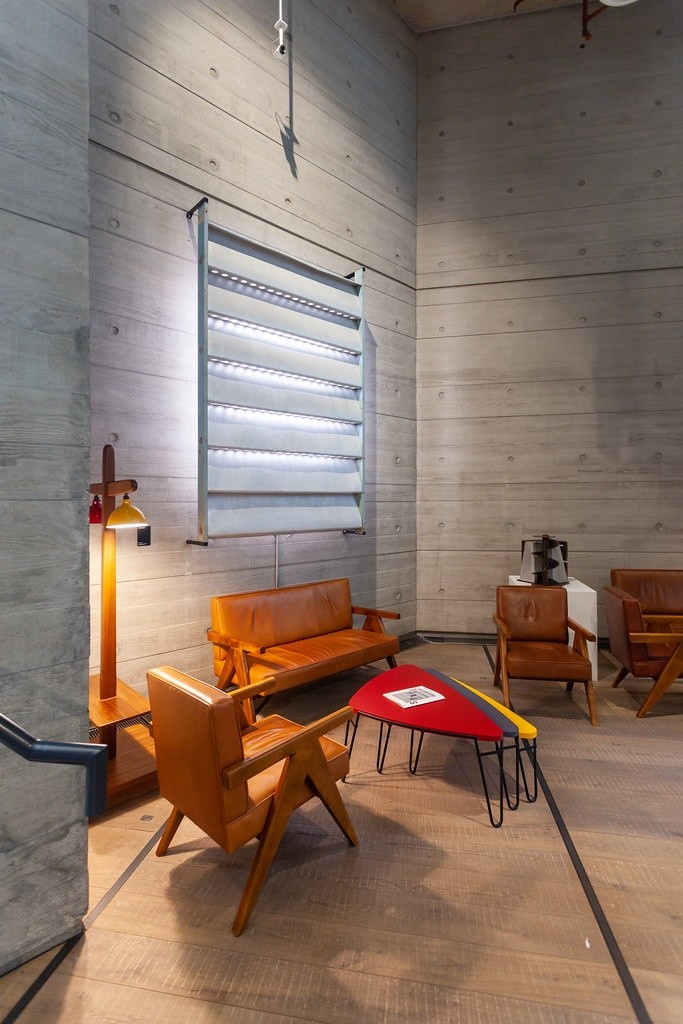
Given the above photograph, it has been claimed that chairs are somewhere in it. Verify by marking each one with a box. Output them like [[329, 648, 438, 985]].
[[145, 666, 357, 939], [493, 585, 599, 726], [602, 585, 683, 718]]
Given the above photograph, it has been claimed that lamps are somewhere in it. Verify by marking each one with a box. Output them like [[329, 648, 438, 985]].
[[105, 494, 147, 529], [89, 495, 102, 524]]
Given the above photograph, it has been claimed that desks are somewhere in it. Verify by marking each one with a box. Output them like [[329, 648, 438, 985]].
[[339, 664, 537, 827], [508, 575, 598, 682]]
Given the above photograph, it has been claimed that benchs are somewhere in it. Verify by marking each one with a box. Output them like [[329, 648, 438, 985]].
[[611, 569, 683, 616], [208, 578, 400, 724]]
